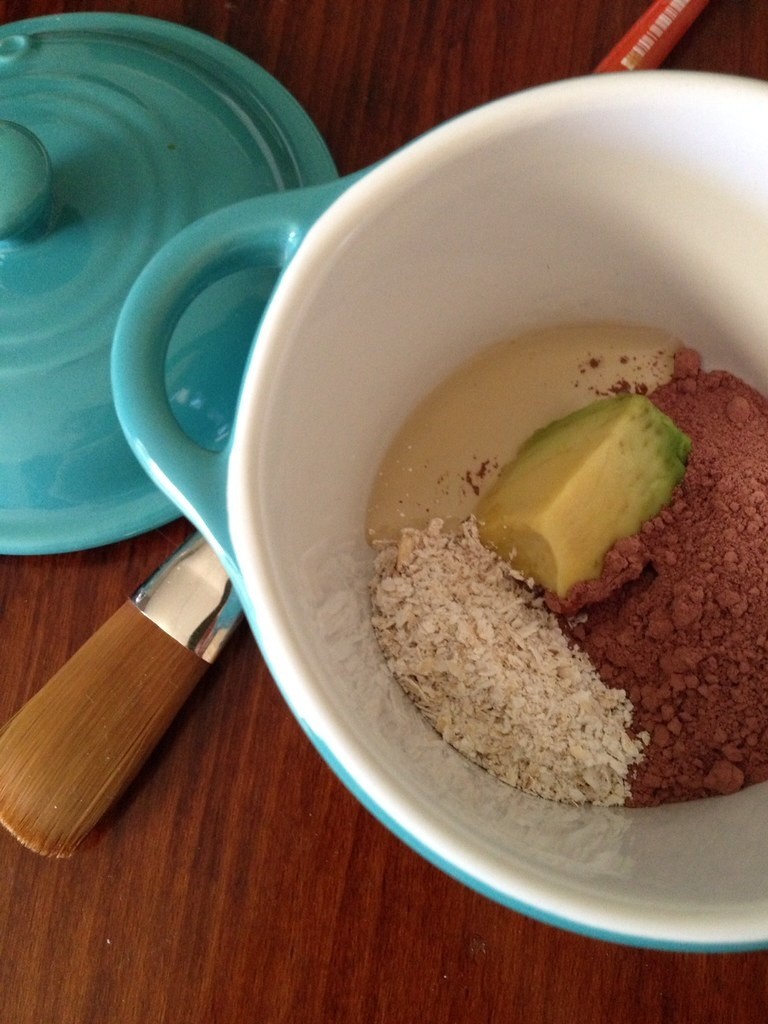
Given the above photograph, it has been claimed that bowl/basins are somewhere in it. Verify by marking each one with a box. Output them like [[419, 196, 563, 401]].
[[114, 69, 768, 954]]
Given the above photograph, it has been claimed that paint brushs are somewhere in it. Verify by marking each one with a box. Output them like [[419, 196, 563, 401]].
[[2, 0, 709, 860]]
